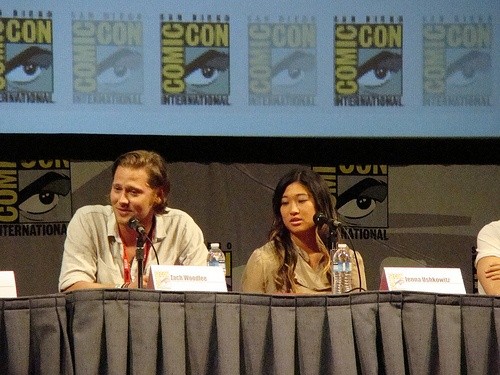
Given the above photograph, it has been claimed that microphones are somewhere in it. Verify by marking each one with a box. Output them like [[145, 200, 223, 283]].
[[313, 212, 342, 227], [128, 217, 146, 234]]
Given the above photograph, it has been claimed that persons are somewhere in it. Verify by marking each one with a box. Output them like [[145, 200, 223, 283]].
[[58, 150, 209, 293], [238, 168, 368, 296], [474, 220, 500, 296]]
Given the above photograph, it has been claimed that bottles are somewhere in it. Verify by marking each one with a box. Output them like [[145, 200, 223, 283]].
[[332, 244, 351, 295], [207, 243, 226, 278]]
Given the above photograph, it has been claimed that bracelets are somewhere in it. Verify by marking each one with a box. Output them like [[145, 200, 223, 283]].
[[116, 282, 132, 288]]
